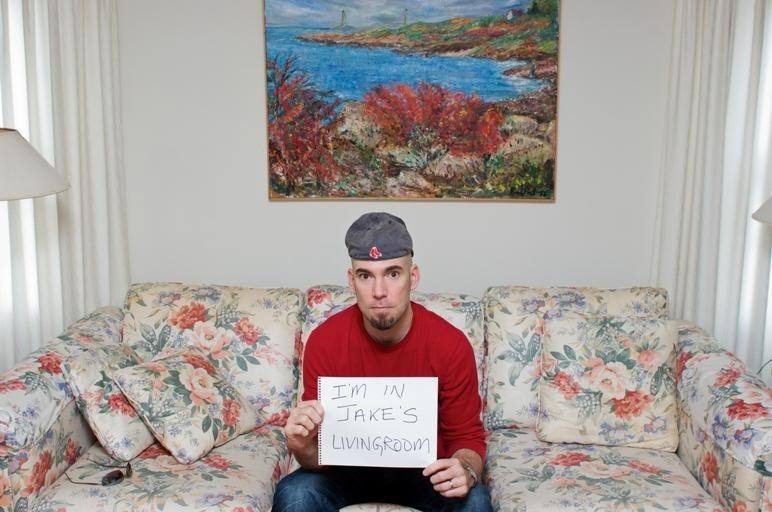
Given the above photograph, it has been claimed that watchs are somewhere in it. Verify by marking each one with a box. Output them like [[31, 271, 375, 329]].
[[461, 462, 478, 488]]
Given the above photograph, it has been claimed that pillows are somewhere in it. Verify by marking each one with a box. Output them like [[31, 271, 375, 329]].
[[536, 309, 679, 453], [296, 285, 483, 432], [481, 286, 669, 431], [120, 284, 304, 427], [112, 345, 266, 465], [60, 343, 156, 463]]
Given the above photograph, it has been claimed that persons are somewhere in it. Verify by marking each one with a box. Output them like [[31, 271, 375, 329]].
[[269, 212, 494, 512]]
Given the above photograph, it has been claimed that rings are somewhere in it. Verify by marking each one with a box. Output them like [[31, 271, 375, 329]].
[[449, 480, 453, 488]]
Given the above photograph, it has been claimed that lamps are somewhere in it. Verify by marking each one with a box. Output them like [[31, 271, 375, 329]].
[[0, 127, 71, 201]]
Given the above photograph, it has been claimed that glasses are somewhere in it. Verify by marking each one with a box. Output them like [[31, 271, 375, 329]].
[[65, 458, 131, 486]]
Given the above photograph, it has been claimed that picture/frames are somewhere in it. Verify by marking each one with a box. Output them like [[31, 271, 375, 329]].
[[261, 0, 562, 203]]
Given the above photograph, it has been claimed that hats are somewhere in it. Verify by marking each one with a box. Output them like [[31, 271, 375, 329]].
[[345, 213, 414, 261]]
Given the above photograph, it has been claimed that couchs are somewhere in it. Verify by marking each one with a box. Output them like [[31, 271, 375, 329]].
[[0, 284, 772, 512]]
